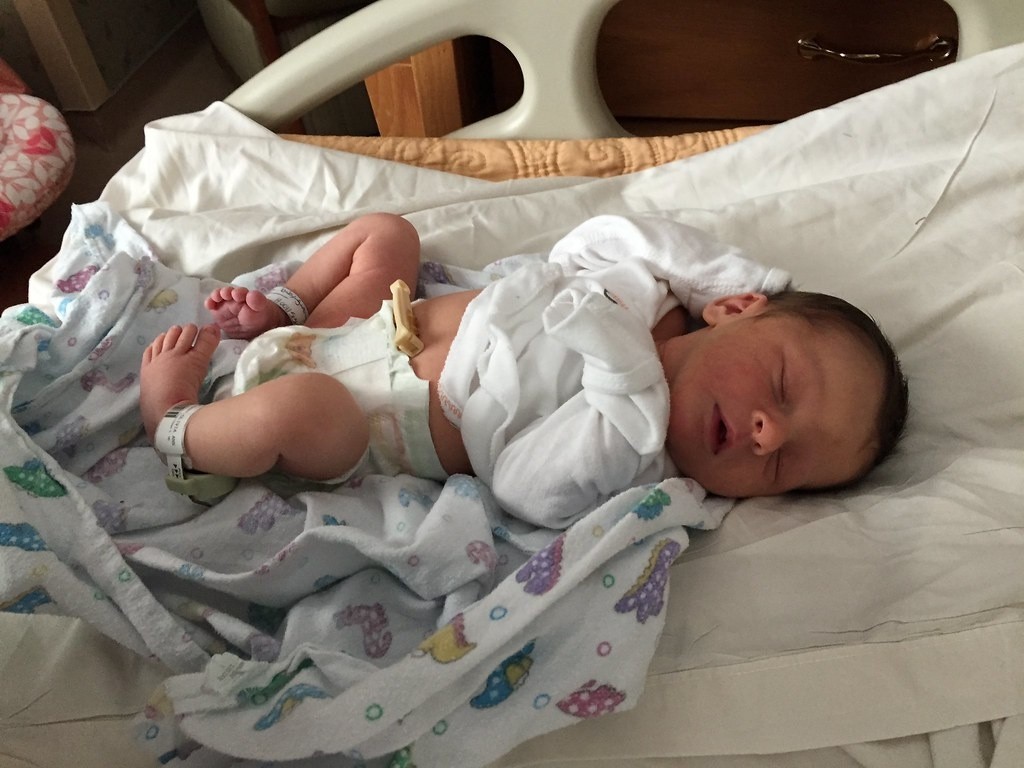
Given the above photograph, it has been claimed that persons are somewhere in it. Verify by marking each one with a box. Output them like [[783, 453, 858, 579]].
[[139, 211, 909, 533]]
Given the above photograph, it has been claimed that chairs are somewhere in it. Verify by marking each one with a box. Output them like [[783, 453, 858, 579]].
[[0, 0, 1024, 768]]
